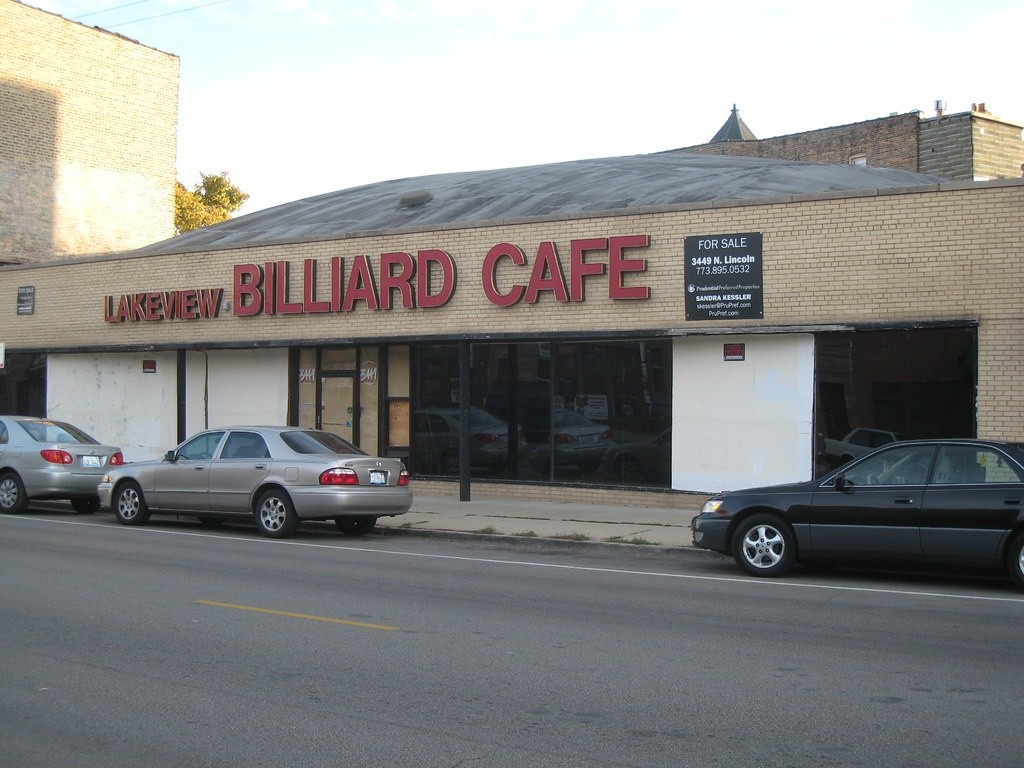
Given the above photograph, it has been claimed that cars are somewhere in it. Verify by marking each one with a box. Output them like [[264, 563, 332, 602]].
[[96, 424, 414, 540], [413, 407, 527, 473], [824, 427, 909, 469], [816, 435, 826, 462], [0, 414, 123, 513], [691, 440, 1024, 586], [602, 428, 672, 482], [552, 408, 616, 471]]
